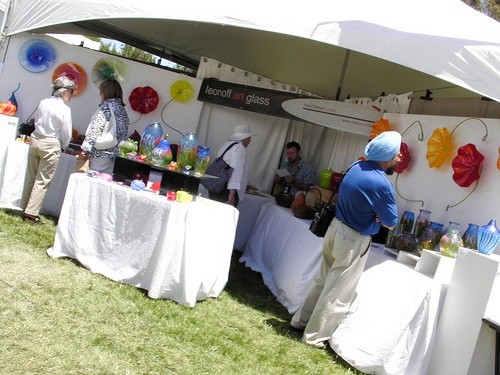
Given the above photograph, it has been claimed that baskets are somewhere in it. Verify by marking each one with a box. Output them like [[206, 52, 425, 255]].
[[295, 204, 315, 219]]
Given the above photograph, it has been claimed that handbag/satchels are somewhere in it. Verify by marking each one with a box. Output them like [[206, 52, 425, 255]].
[[18, 119, 35, 136], [309, 206, 334, 238], [199, 142, 239, 195], [93, 102, 117, 150]]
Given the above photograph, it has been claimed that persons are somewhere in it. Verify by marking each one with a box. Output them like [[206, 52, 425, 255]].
[[277, 141, 317, 196], [290, 131, 402, 350], [20, 76, 76, 223], [209, 124, 259, 208], [80, 79, 129, 175]]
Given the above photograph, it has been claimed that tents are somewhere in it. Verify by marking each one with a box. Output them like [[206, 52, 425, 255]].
[[0, 0, 500, 195]]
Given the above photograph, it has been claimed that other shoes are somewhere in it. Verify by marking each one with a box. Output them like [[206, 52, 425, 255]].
[[289, 324, 304, 332], [318, 342, 330, 349]]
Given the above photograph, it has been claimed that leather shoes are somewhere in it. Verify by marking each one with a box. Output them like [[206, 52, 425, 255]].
[[23, 213, 47, 225]]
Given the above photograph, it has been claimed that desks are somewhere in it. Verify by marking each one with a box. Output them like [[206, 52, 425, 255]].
[[112, 154, 220, 201], [233, 190, 275, 256], [46, 173, 239, 308], [239, 201, 449, 375], [0, 140, 77, 218]]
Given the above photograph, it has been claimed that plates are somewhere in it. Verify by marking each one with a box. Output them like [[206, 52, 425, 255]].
[[18, 38, 127, 98]]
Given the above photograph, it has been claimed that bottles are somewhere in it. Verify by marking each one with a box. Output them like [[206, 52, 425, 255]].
[[118, 137, 138, 159], [439, 224, 464, 258], [477, 219, 500, 255], [193, 145, 211, 176], [462, 223, 480, 250], [152, 139, 172, 168], [442, 221, 461, 235], [385, 223, 403, 247], [413, 209, 432, 239], [177, 131, 198, 172], [415, 226, 438, 257], [139, 121, 163, 160], [399, 210, 414, 234], [395, 232, 416, 253], [428, 221, 444, 244]]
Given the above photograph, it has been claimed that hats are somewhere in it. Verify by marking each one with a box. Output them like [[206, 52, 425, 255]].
[[225, 124, 259, 141], [365, 131, 402, 161], [51, 75, 77, 97]]
